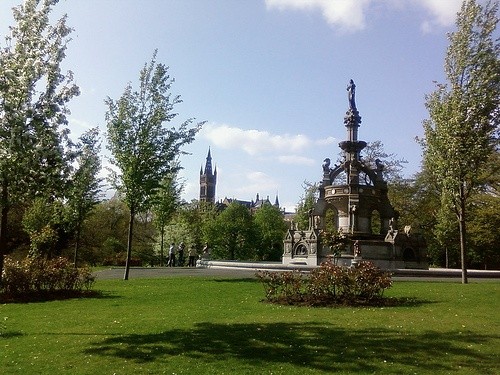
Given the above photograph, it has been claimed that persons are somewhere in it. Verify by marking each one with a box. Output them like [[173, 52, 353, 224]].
[[168, 243, 177, 267], [187, 246, 196, 267], [178, 242, 186, 267]]
